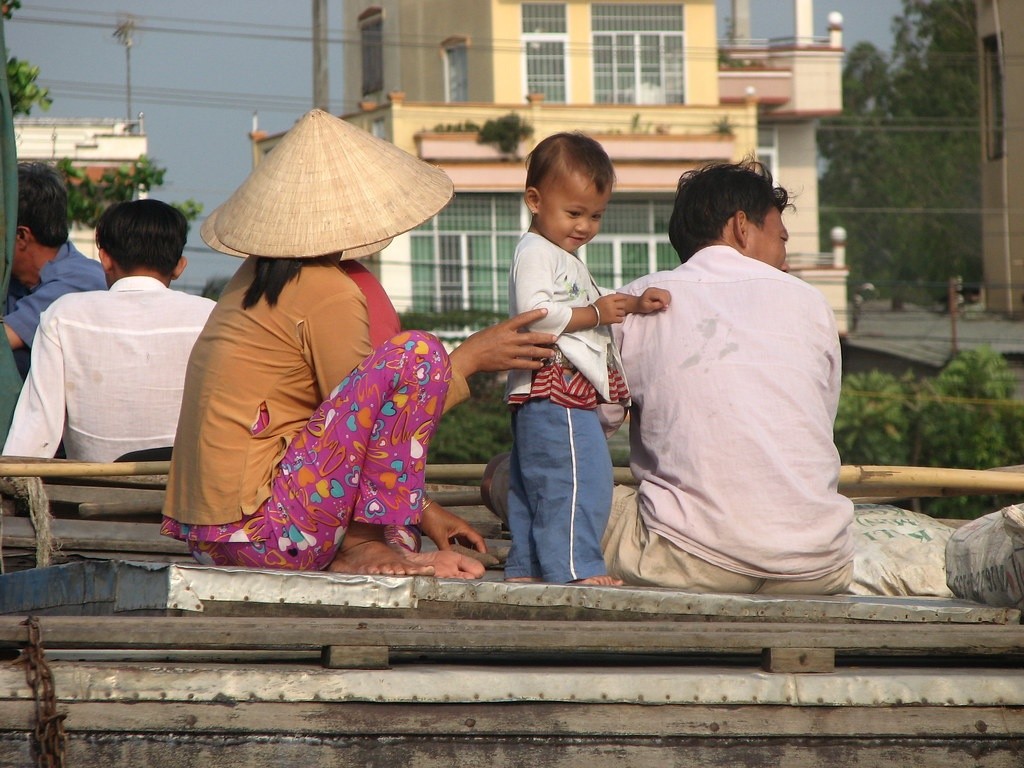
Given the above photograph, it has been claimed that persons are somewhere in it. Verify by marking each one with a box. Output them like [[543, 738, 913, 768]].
[[479, 156, 856, 595], [3, 161, 109, 458], [158, 109, 559, 582], [502, 132, 671, 586], [0, 199, 219, 524]]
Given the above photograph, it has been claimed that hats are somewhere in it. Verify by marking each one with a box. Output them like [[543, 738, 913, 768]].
[[201, 202, 392, 262], [214, 108, 454, 258]]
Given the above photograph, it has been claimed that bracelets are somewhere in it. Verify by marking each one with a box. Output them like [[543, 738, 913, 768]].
[[586, 304, 600, 330], [421, 492, 433, 512]]
